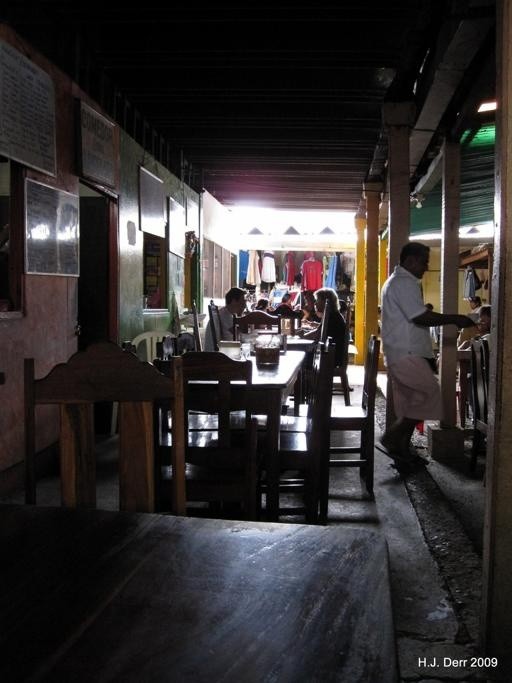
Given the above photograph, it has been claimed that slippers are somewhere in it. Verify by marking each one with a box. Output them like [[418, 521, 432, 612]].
[[375, 441, 430, 476]]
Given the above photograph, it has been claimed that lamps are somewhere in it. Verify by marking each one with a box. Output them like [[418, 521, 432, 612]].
[[413, 193, 426, 209]]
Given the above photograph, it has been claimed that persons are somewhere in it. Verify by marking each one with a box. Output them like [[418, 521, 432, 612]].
[[375, 243, 476, 467], [203, 287, 491, 366]]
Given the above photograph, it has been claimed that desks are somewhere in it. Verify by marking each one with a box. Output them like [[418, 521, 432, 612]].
[[0, 503, 399, 683]]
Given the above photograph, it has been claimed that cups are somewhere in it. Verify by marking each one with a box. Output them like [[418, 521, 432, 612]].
[[240, 346, 250, 360], [248, 323, 254, 333]]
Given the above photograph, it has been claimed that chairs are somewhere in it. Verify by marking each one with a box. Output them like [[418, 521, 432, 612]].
[[23, 299, 489, 529]]
